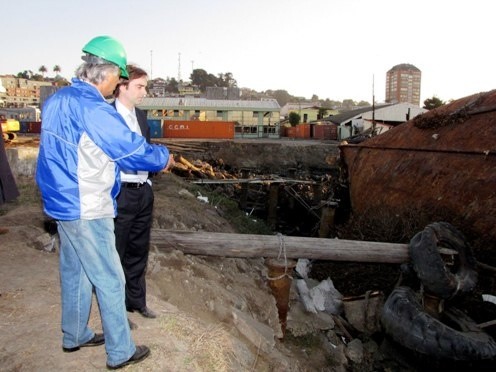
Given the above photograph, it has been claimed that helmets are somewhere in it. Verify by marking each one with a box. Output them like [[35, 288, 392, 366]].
[[81, 36, 129, 79]]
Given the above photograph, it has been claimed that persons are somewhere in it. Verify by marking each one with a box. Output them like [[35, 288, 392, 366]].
[[106, 65, 157, 328], [37, 35, 174, 370]]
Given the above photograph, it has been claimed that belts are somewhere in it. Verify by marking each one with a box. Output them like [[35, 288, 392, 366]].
[[119, 181, 147, 188]]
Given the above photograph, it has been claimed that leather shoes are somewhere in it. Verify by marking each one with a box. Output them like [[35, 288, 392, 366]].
[[126, 304, 156, 317], [61, 334, 106, 352], [104, 346, 150, 369]]
